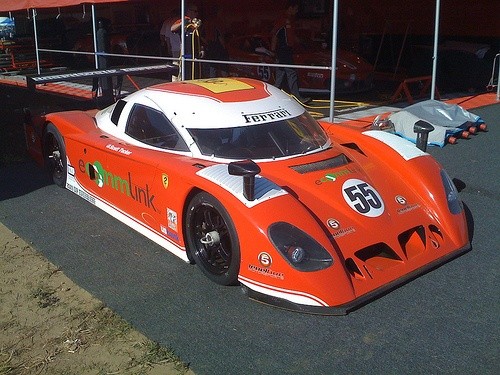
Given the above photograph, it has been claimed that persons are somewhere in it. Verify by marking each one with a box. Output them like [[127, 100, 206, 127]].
[[272, 13, 312, 104], [172, 2, 204, 82], [160, 8, 183, 70]]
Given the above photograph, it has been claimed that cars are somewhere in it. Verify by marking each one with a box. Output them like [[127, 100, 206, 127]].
[[38, 75, 472, 315], [224, 34, 373, 98]]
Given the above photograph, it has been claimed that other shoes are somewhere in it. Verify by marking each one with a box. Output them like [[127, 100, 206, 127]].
[[300, 97, 312, 104]]
